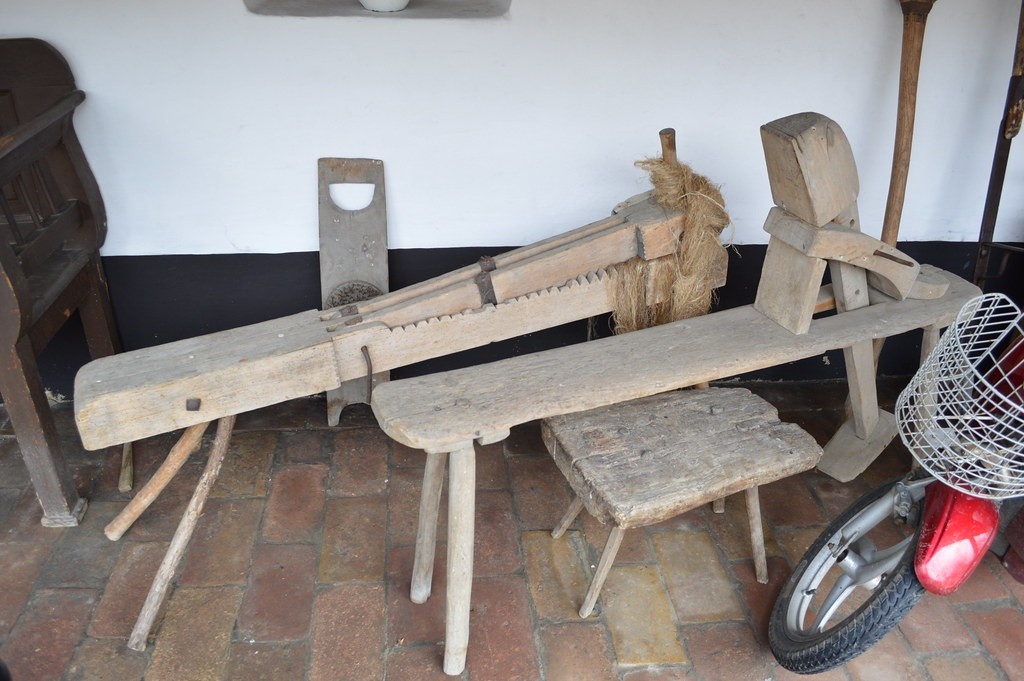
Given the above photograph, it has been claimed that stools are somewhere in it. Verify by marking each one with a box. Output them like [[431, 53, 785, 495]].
[[541, 386, 825, 619]]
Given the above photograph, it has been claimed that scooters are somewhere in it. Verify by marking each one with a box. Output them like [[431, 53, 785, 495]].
[[767, 262, 1024, 676]]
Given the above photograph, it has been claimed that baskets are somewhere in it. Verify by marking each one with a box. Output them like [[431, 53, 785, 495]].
[[895, 293, 1024, 499]]
